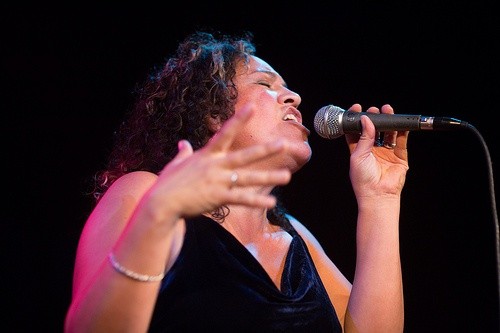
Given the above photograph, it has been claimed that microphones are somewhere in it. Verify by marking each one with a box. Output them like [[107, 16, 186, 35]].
[[314, 105, 468, 139]]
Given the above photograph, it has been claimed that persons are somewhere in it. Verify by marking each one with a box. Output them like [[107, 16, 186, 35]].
[[65, 34, 409, 333]]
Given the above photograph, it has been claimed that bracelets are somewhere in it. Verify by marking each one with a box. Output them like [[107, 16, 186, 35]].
[[106, 252, 165, 281]]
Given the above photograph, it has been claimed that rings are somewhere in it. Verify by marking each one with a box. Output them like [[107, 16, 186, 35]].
[[382, 141, 396, 149], [231, 170, 238, 187]]
